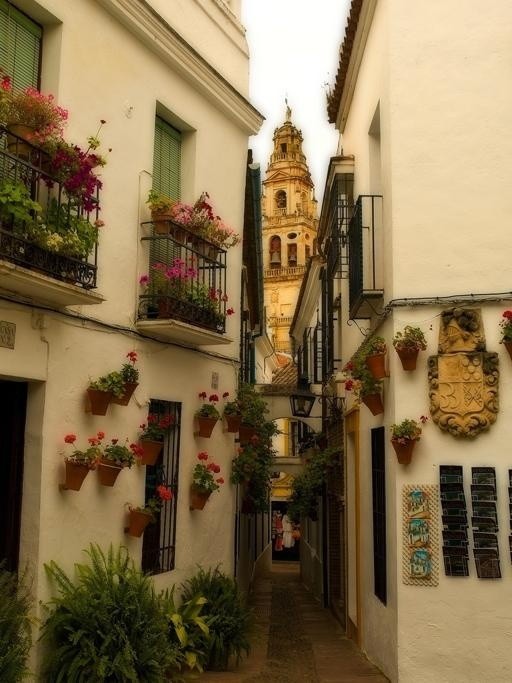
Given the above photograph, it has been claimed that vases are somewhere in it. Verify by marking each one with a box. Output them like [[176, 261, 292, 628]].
[[126, 509, 152, 539], [390, 438, 414, 465]]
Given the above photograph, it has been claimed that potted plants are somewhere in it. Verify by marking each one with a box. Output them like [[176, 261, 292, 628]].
[[32, 538, 260, 683], [285, 446, 345, 525]]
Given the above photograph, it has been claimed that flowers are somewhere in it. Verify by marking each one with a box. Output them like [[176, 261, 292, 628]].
[[0, 72, 237, 324], [341, 308, 512, 446], [58, 347, 260, 514]]
